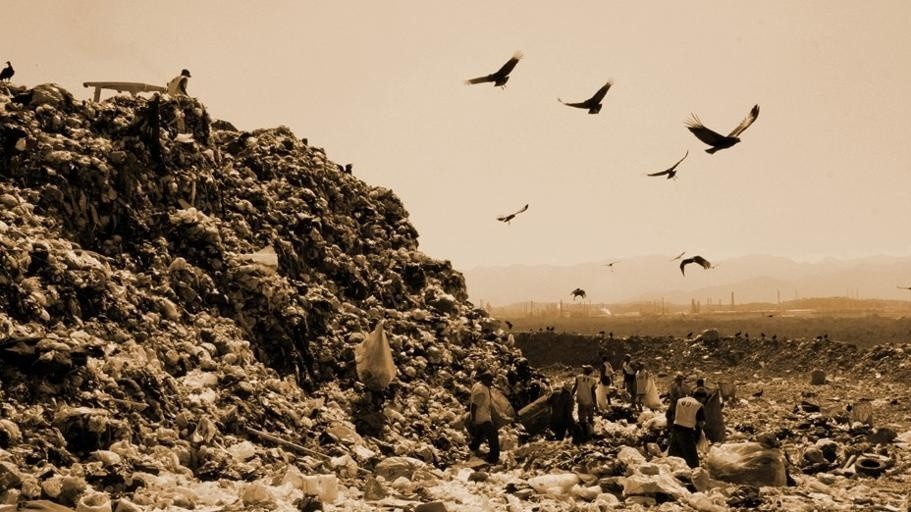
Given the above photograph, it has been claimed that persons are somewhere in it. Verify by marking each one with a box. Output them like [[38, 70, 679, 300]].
[[570, 353, 718, 469], [469, 370, 500, 463], [167, 69, 192, 98]]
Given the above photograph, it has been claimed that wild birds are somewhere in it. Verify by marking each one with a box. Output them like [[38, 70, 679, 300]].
[[753, 388, 764, 398], [496, 204, 529, 222], [10, 89, 35, 106], [0, 61, 14, 83], [466, 50, 523, 90], [557, 81, 613, 114], [682, 104, 760, 154], [603, 261, 618, 267], [648, 151, 688, 179], [680, 256, 711, 276], [570, 288, 586, 301], [673, 251, 686, 260]]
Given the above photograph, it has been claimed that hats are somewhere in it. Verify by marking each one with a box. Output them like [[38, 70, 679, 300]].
[[182, 69, 191, 77]]
